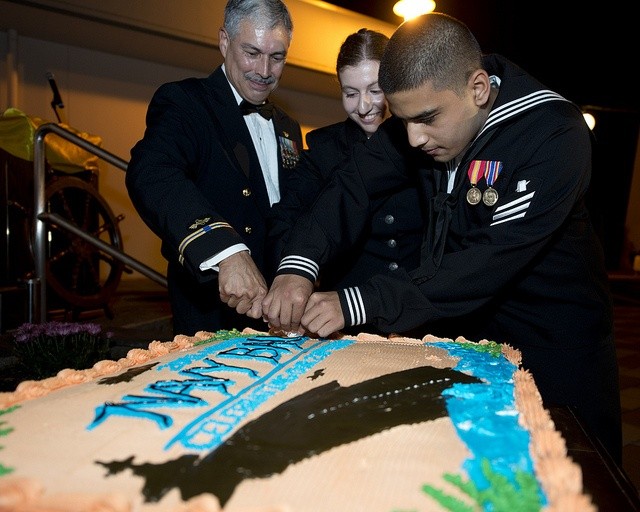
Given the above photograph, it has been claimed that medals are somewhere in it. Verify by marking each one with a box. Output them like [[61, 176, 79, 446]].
[[483, 161, 503, 206], [466, 160, 487, 204]]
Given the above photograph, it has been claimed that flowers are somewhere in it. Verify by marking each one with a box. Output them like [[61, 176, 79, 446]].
[[10, 320, 108, 358]]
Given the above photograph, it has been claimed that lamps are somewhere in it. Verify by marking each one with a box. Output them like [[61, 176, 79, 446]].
[[393, 0, 436, 22]]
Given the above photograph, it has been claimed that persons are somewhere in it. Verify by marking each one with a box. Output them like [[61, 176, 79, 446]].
[[126, 0, 304, 341], [262, 13, 624, 467], [262, 28, 431, 336]]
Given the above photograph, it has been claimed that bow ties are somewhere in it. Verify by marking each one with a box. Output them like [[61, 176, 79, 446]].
[[238, 99, 273, 121]]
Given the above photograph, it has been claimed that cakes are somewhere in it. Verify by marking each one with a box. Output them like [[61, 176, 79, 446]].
[[0, 327, 596, 508]]
